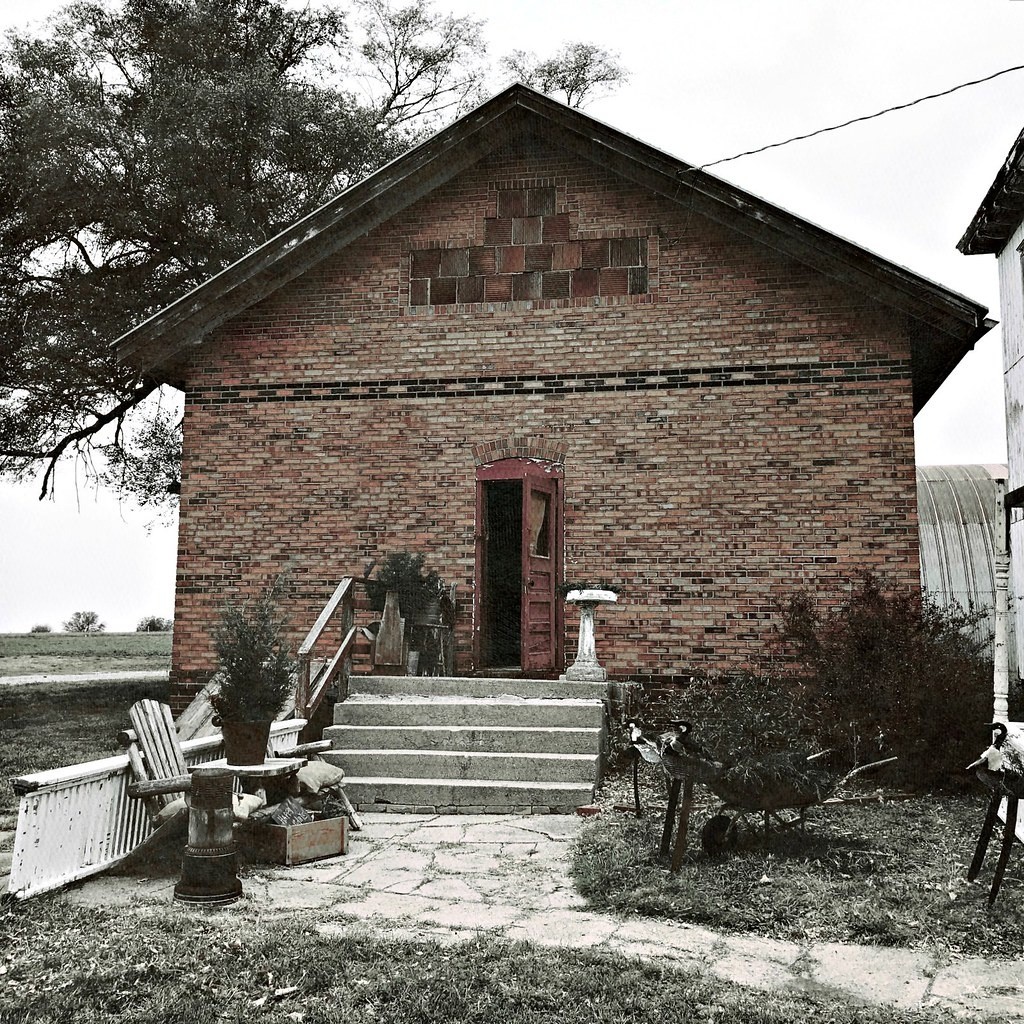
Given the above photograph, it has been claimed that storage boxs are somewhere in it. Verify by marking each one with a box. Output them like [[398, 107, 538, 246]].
[[250, 815, 350, 869]]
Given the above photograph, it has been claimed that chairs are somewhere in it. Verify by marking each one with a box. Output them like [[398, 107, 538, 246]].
[[106, 698, 192, 878], [266, 737, 364, 830]]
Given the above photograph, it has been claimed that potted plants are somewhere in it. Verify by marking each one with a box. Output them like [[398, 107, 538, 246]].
[[413, 571, 444, 625], [201, 593, 306, 766]]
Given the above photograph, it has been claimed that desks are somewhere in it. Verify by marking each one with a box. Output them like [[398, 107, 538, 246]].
[[186, 756, 309, 804]]
